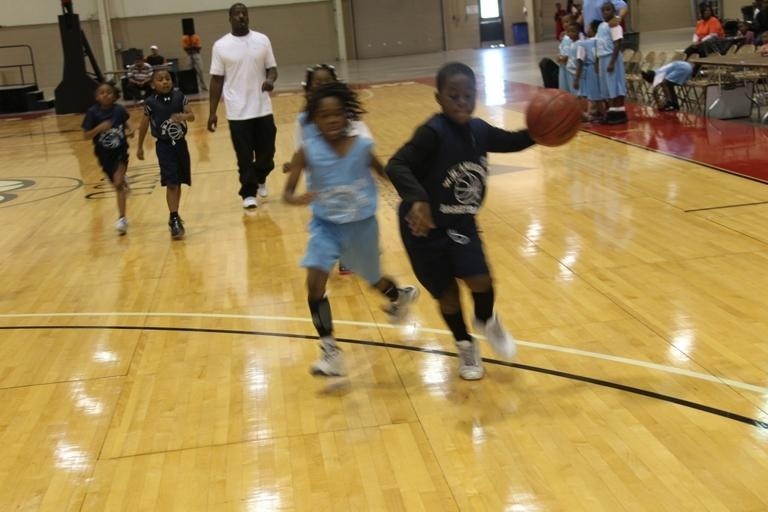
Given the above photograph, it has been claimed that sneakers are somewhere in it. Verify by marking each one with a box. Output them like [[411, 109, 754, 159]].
[[314, 343, 343, 374], [116, 217, 127, 232], [244, 195, 258, 208], [258, 182, 267, 196], [455, 335, 484, 380], [388, 285, 418, 318], [169, 216, 184, 236], [472, 311, 514, 356]]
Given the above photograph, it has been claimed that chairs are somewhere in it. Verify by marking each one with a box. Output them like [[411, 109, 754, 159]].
[[621, 44, 768, 123]]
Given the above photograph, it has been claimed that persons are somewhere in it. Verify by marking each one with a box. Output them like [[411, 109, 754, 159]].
[[283, 63, 386, 274], [385, 63, 536, 382], [283, 82, 420, 379], [553, 0, 768, 126], [80, 32, 207, 239], [208, 2, 277, 210]]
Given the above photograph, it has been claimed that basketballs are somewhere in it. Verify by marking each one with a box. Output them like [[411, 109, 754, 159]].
[[527, 89, 585, 146]]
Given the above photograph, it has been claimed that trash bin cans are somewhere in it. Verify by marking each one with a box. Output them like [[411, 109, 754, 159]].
[[511, 22, 528, 45]]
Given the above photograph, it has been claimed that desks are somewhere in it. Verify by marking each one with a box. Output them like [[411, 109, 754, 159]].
[[120, 67, 199, 101]]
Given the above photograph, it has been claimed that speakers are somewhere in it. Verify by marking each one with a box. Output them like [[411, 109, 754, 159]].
[[182, 18, 194, 36]]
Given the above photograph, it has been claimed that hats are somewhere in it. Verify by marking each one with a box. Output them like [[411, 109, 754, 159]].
[[150, 45, 158, 50]]
